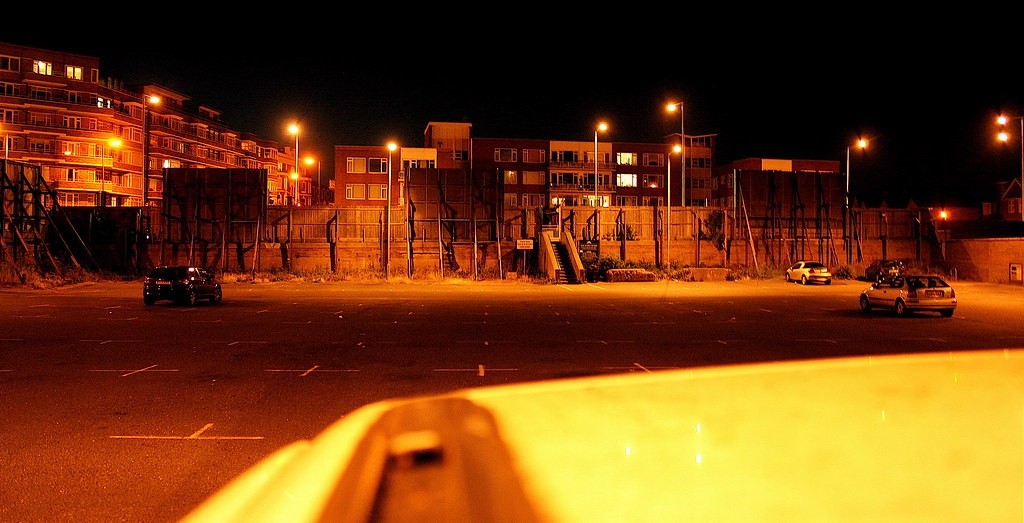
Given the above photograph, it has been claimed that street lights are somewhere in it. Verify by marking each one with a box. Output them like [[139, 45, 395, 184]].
[[594, 120, 608, 239], [101, 137, 122, 206], [846, 134, 868, 263], [386, 141, 398, 277], [667, 145, 682, 266], [288, 123, 301, 206], [995, 114, 1024, 289], [666, 101, 687, 206], [143, 95, 161, 251]]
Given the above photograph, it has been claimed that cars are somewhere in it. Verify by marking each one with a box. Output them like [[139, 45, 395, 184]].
[[860, 273, 959, 319], [144, 266, 222, 307], [785, 261, 832, 286]]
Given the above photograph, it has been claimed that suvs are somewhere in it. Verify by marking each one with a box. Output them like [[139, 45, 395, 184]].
[[865, 257, 907, 289]]
